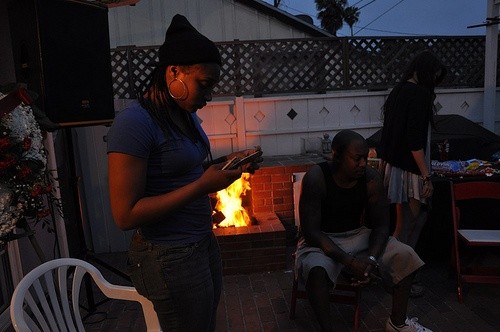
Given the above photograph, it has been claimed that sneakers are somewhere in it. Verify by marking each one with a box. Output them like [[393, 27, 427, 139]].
[[385, 312, 434, 332]]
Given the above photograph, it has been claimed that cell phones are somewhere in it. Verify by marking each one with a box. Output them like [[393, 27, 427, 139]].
[[232, 150, 264, 169]]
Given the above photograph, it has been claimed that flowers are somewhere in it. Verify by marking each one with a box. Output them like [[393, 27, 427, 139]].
[[0, 83, 70, 246]]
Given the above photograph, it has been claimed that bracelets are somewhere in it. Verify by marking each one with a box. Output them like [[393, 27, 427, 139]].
[[348, 256, 355, 268]]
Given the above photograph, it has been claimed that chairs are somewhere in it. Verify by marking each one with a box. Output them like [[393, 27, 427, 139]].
[[449, 181, 500, 304], [10, 258, 162, 332], [290, 172, 362, 332]]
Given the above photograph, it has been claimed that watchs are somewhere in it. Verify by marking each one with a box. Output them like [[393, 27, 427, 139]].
[[423, 176, 431, 182], [367, 255, 377, 264]]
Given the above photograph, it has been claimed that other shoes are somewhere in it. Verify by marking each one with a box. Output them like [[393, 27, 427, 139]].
[[408, 284, 426, 297]]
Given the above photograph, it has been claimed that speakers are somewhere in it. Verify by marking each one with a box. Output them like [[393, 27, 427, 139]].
[[8, 0, 115, 130]]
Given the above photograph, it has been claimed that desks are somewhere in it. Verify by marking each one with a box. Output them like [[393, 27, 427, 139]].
[[430, 173, 500, 277]]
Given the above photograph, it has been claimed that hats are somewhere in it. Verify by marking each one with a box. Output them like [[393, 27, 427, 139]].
[[158, 13, 221, 67]]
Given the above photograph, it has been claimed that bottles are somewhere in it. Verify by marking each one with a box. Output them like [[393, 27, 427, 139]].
[[368, 146, 377, 171], [321, 134, 332, 153]]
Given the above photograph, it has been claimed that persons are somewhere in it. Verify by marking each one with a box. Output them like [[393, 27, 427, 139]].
[[106, 12, 263, 332], [379, 52, 448, 332], [294, 131, 433, 332]]
